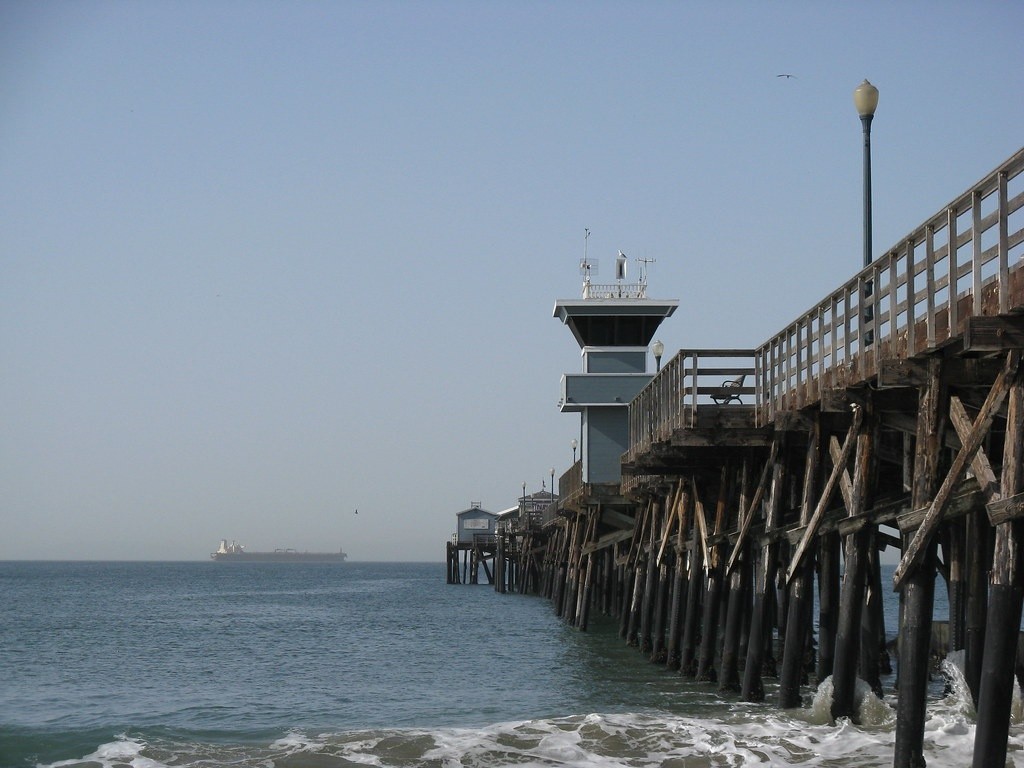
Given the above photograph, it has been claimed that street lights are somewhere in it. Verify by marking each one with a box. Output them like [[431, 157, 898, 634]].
[[571, 438, 578, 465], [550, 466, 555, 504], [652, 339, 664, 374], [522, 480, 527, 511], [853, 78, 881, 349]]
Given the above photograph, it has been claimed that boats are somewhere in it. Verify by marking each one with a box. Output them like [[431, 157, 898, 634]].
[[210, 539, 348, 563]]
